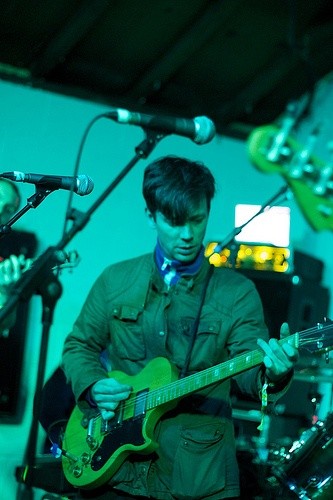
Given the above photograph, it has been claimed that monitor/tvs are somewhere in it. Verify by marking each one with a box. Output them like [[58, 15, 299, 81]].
[[235, 203, 290, 248]]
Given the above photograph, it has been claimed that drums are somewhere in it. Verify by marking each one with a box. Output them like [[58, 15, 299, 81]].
[[272, 424, 333, 500]]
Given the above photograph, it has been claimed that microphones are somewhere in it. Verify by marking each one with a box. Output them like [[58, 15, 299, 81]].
[[2, 171, 94, 196], [105, 108, 216, 145]]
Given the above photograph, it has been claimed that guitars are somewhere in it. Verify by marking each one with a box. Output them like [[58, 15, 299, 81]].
[[62, 316, 333, 490]]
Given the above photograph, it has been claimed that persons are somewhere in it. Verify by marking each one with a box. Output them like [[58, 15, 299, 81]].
[[61, 155, 298, 500], [1, 178, 37, 344]]
